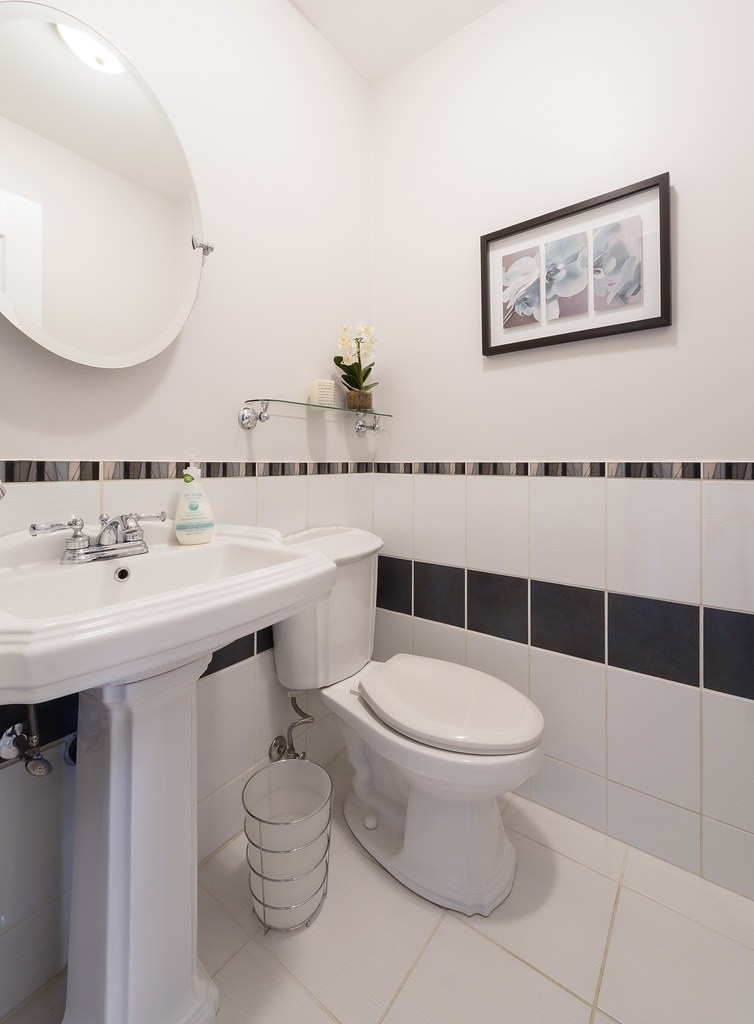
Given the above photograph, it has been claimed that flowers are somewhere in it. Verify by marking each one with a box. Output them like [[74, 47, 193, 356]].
[[333, 322, 378, 390]]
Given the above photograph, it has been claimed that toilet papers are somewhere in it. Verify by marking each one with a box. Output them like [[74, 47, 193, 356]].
[[311, 379, 336, 407]]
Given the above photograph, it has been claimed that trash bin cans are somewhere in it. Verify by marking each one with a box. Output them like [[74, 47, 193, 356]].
[[241, 758, 335, 933]]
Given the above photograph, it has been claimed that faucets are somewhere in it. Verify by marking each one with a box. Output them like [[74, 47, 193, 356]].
[[97, 514, 142, 544]]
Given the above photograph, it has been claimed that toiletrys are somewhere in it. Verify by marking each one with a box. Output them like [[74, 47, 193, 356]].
[[173, 453, 216, 544]]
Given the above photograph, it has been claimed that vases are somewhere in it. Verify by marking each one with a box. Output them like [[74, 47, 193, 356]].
[[346, 389, 373, 412]]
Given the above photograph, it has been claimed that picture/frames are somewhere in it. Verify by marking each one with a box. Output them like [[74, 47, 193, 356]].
[[479, 171, 671, 357]]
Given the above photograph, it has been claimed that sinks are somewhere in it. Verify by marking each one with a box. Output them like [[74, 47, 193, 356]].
[[0, 519, 337, 703]]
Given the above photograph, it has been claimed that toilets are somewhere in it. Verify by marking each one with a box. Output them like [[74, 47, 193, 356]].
[[281, 526, 545, 918]]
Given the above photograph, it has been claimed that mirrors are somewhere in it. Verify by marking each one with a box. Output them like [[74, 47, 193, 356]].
[[0, 1, 209, 371]]
